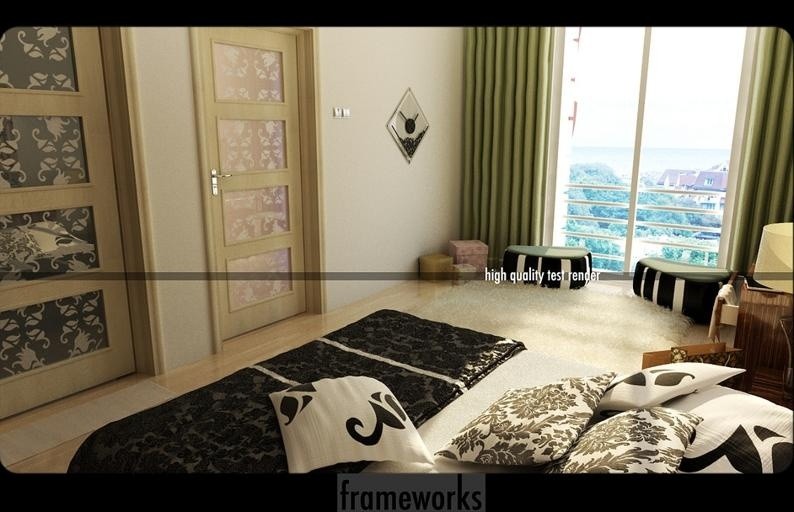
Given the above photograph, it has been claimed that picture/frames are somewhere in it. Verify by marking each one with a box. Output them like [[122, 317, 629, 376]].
[[388, 88, 429, 164]]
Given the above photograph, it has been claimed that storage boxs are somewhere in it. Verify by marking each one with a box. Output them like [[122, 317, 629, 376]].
[[450, 239, 488, 278], [418, 254, 452, 283]]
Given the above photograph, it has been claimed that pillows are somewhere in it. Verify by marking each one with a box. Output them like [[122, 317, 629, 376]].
[[542, 407, 704, 473], [599, 362, 746, 407], [270, 375, 431, 473], [434, 372, 618, 463], [657, 385, 794, 475]]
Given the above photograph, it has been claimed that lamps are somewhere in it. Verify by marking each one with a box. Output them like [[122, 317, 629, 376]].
[[754, 221, 794, 293]]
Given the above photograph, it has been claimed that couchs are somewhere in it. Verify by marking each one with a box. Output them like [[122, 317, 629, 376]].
[[633, 259, 731, 321], [506, 246, 591, 290]]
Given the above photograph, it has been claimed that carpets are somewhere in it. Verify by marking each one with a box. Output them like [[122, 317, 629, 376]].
[[360, 281, 689, 473]]
